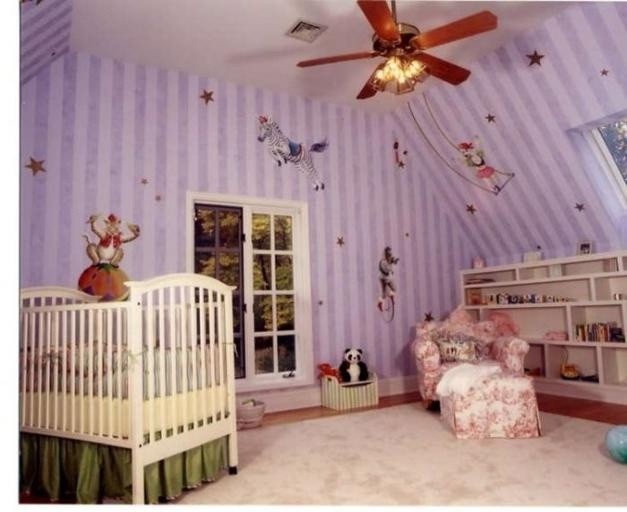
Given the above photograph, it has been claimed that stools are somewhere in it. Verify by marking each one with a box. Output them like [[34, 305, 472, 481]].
[[440, 372, 541, 439]]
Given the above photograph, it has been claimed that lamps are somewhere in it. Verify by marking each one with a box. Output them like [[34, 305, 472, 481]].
[[371, 0, 431, 97]]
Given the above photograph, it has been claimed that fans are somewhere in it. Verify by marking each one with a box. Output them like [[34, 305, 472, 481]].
[[295, 1, 500, 99]]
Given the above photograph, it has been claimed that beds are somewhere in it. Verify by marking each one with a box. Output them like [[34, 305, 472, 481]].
[[20, 270, 239, 505]]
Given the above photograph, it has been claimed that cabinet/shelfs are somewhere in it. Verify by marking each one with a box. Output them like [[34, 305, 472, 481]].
[[458, 249, 627, 425]]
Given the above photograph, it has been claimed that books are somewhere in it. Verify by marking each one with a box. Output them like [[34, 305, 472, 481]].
[[572, 321, 617, 341]]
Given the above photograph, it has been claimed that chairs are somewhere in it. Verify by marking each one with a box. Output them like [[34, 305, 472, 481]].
[[412, 318, 531, 412]]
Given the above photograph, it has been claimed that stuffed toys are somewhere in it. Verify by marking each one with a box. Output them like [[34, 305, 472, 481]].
[[339, 349, 368, 382]]
